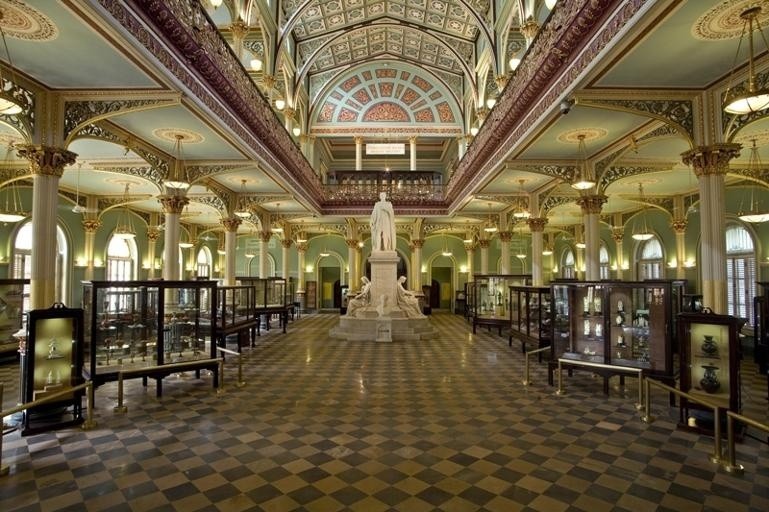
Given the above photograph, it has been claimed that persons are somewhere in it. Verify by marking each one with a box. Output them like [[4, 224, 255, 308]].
[[369, 192, 397, 252], [377, 296, 393, 315], [398, 276, 423, 314], [347, 276, 372, 314]]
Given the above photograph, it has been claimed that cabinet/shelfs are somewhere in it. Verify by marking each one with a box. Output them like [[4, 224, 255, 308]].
[[677, 309, 749, 448], [0, 280, 300, 439], [554, 281, 684, 412], [448, 280, 557, 369]]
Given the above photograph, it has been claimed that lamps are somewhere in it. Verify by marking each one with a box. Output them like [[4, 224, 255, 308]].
[[720, 6, 769, 117], [292, 126, 302, 137], [508, 53, 521, 71], [210, 0, 223, 10], [486, 97, 498, 108], [630, 183, 655, 241], [440, 184, 587, 258], [738, 141, 769, 225], [545, 1, 556, 9], [0, 141, 28, 227], [570, 138, 597, 190], [250, 55, 263, 71], [162, 136, 192, 189], [275, 97, 286, 110], [178, 206, 195, 248], [114, 184, 138, 241], [213, 180, 332, 259]]
